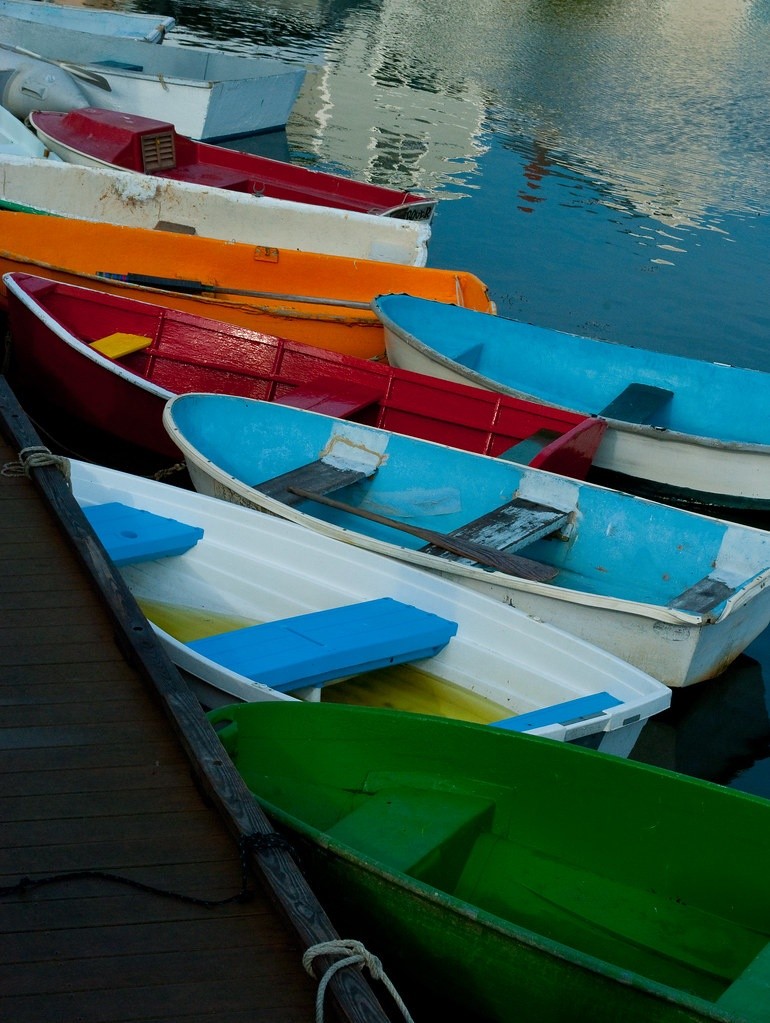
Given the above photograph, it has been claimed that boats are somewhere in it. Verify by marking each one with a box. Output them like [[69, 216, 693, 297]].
[[0, 156, 432, 267], [0, 0, 306, 162], [62, 457, 672, 760], [3, 272, 608, 481], [0, 210, 497, 366], [370, 292, 770, 513], [163, 391, 770, 687], [30, 108, 439, 224], [205, 702, 770, 1023]]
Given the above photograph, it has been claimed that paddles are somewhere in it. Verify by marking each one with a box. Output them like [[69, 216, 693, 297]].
[[287, 486, 560, 583], [1, 40, 113, 92]]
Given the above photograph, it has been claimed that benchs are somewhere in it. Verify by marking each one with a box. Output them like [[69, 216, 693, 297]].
[[276, 376, 383, 419], [596, 383, 675, 426], [81, 502, 204, 565], [713, 942, 770, 1023], [490, 691, 625, 733], [180, 597, 458, 694], [319, 786, 498, 874], [496, 427, 565, 466], [665, 568, 753, 614], [186, 167, 250, 190], [250, 455, 379, 506], [89, 332, 153, 359], [417, 496, 575, 571]]
